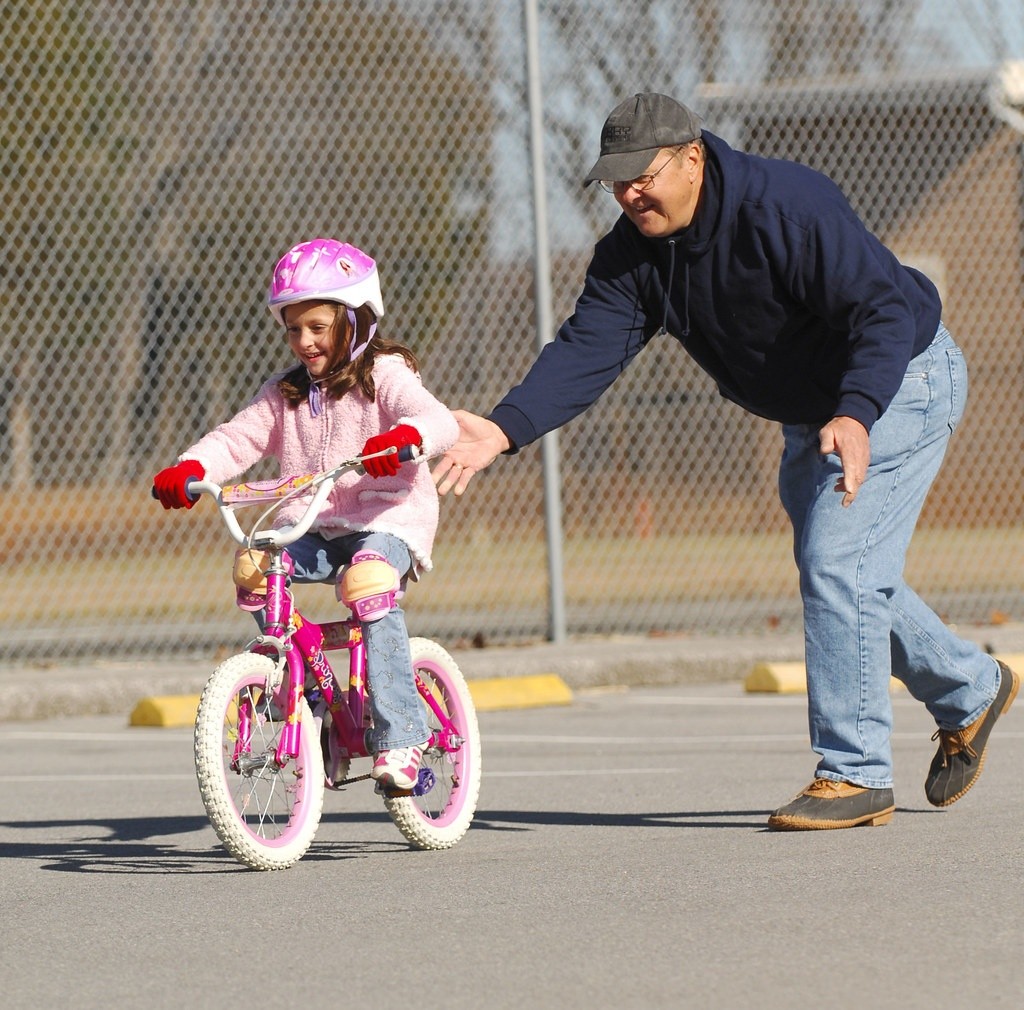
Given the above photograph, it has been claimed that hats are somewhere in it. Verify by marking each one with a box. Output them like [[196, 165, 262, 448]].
[[582, 92, 702, 189]]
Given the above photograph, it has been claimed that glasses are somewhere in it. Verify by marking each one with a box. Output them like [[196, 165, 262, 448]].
[[598, 145, 685, 193]]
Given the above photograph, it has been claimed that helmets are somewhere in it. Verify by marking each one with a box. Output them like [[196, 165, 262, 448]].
[[269, 238, 386, 317]]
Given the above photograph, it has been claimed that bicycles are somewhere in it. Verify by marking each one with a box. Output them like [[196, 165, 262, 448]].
[[151, 442, 485, 872]]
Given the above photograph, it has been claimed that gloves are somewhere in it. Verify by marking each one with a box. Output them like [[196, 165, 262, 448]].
[[153, 459, 205, 509], [364, 425, 421, 478]]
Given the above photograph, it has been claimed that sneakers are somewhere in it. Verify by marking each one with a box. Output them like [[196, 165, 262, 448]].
[[370, 742, 430, 789], [254, 693, 283, 722], [924, 659, 1021, 807], [769, 773, 898, 830]]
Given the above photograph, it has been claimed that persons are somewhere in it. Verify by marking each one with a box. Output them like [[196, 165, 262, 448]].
[[432, 92, 1021, 827], [153, 238, 461, 790]]
